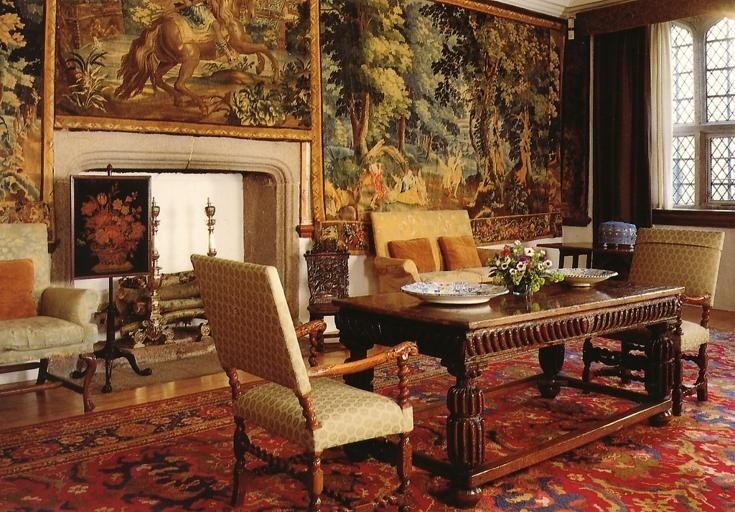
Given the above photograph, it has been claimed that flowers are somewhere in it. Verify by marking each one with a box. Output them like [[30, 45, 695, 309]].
[[486, 239, 565, 293]]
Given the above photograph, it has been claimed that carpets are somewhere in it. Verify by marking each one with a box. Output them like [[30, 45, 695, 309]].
[[1, 326, 735, 512]]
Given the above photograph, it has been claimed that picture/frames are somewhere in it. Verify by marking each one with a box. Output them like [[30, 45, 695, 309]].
[[69, 175, 152, 280]]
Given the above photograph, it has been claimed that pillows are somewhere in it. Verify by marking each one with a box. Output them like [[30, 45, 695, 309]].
[[388, 238, 436, 273], [0, 259, 37, 321], [438, 232, 482, 271]]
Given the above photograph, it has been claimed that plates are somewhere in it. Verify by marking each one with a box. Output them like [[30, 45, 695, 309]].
[[547, 268, 618, 287], [400, 281, 509, 306]]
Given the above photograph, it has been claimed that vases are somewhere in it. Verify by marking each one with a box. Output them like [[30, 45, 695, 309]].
[[513, 284, 529, 294]]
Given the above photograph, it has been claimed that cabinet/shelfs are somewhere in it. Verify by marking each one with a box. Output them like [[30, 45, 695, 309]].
[[594, 246, 634, 281]]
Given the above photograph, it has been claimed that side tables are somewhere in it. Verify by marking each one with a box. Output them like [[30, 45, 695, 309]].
[[537, 242, 593, 269]]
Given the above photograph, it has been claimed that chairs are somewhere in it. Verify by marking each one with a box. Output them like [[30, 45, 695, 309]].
[[303, 237, 351, 351], [0, 223, 99, 413], [190, 255, 419, 511], [582, 227, 724, 418]]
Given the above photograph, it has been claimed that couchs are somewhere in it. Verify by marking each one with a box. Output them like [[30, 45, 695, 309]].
[[370, 210, 504, 294]]
